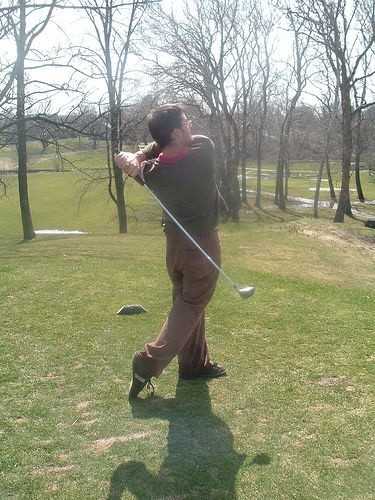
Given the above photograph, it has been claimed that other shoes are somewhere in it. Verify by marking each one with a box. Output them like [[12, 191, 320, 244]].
[[179, 361, 226, 377], [129, 352, 151, 398]]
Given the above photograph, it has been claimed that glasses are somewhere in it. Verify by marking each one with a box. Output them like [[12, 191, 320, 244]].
[[178, 119, 192, 129]]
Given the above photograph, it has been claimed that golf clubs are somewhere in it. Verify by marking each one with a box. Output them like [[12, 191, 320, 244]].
[[113, 148, 256, 299]]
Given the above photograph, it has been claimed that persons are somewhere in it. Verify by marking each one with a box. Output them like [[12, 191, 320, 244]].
[[114, 104, 228, 399]]
